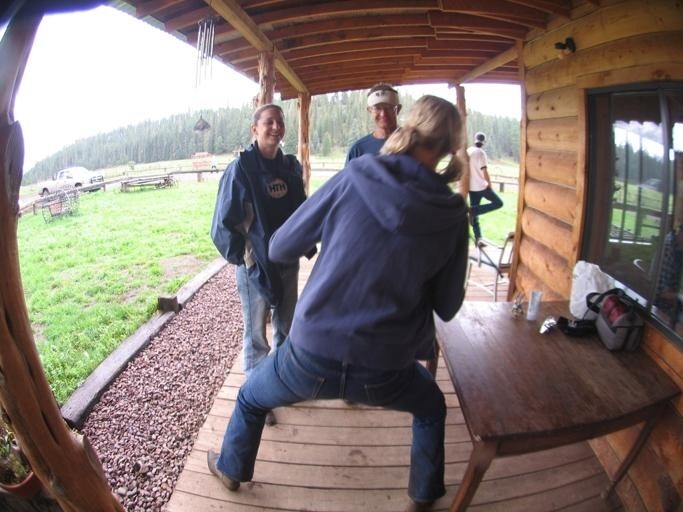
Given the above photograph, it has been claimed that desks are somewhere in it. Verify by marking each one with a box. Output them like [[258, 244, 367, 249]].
[[427, 300, 681, 511]]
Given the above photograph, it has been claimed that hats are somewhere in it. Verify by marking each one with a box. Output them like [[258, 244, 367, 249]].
[[366, 90, 400, 107], [475, 133, 485, 144]]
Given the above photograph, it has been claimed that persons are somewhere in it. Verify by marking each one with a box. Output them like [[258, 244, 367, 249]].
[[208, 101, 318, 427], [204, 93, 472, 512], [463, 130, 503, 250], [343, 81, 403, 169]]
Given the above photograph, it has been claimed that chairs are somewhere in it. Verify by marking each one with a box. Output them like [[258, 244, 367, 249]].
[[465, 231, 515, 300]]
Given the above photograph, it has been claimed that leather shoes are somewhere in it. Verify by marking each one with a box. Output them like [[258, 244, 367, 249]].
[[207, 449, 240, 490]]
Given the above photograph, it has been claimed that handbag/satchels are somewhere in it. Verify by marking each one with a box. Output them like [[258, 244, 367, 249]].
[[569, 261, 615, 321], [596, 294, 643, 353]]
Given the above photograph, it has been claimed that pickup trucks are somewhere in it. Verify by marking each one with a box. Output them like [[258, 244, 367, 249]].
[[37, 166, 105, 199]]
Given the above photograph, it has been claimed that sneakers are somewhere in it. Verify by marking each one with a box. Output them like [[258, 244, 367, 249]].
[[477, 238, 488, 247], [469, 207, 475, 225]]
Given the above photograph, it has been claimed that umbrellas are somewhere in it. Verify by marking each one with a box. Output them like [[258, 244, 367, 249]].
[[192, 114, 211, 151]]
